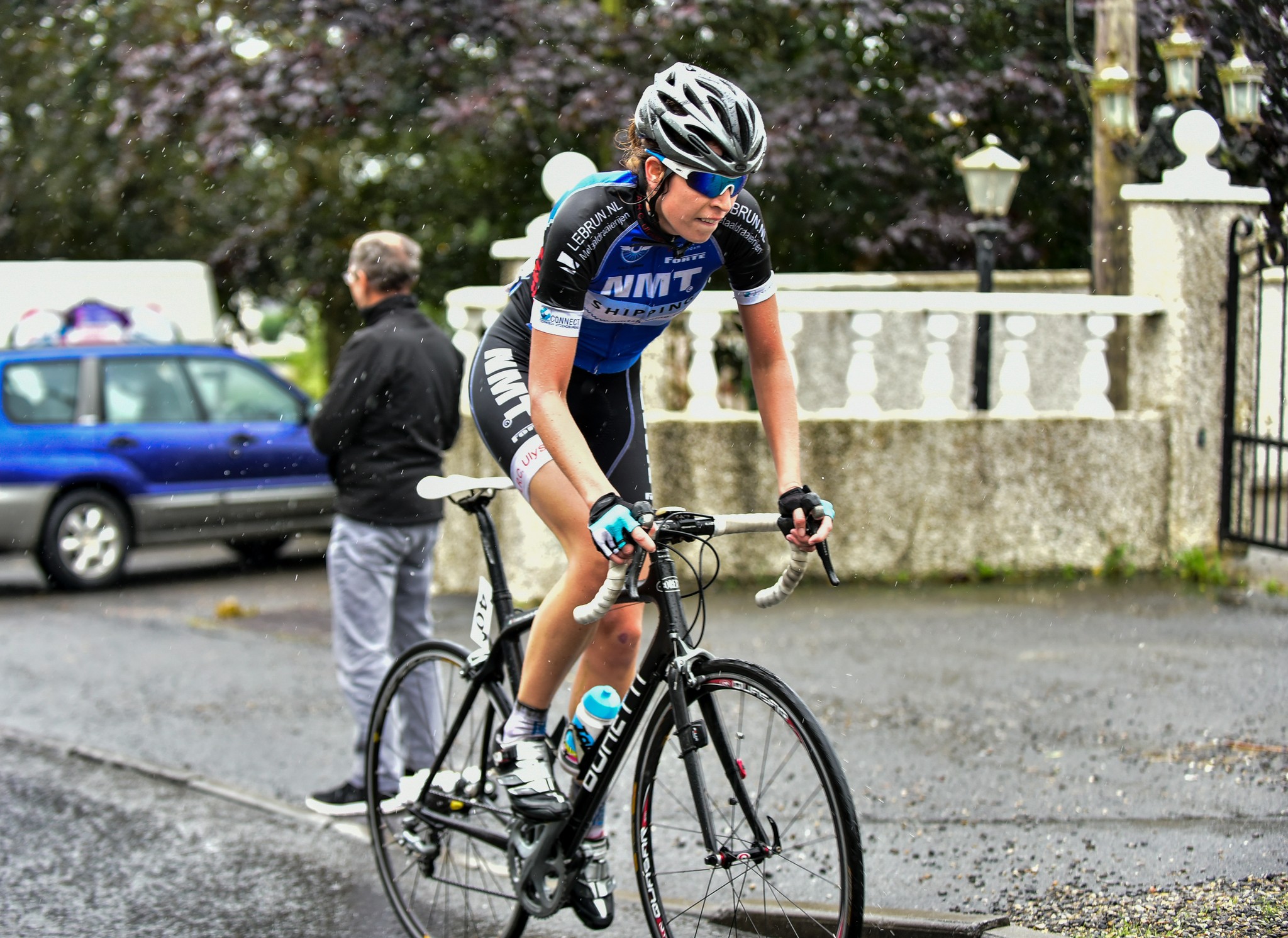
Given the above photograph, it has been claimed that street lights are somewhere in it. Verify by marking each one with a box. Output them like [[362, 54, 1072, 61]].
[[944, 129, 1034, 412]]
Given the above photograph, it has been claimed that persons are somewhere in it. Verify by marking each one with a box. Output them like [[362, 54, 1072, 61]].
[[304, 232, 465, 828], [474, 63, 834, 930]]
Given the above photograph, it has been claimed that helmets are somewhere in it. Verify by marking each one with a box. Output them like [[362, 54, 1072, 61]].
[[634, 61, 767, 176]]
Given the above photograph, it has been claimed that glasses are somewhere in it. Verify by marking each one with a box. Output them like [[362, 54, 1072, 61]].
[[642, 147, 749, 199], [342, 271, 360, 286]]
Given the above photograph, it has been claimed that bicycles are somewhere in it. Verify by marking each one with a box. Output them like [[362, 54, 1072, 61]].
[[360, 473, 866, 938]]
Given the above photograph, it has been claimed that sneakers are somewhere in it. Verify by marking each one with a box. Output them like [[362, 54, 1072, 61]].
[[306, 780, 405, 817], [491, 724, 573, 821], [569, 839, 616, 930]]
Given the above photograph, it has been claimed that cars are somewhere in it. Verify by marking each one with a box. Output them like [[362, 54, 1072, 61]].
[[1, 340, 340, 595]]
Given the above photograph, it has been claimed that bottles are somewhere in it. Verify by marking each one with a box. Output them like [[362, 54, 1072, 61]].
[[556, 684, 621, 778]]
